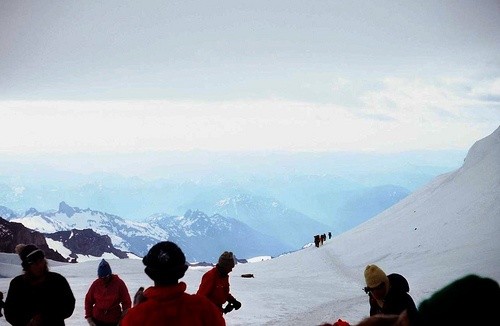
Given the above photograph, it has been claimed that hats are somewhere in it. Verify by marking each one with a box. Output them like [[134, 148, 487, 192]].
[[143, 241, 186, 274], [365, 264, 389, 289], [16, 244, 44, 262], [98, 258, 111, 275], [219, 251, 235, 270]]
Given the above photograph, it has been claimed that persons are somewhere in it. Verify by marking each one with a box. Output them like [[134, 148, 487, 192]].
[[117, 241, 226, 326], [0, 292, 5, 317], [85, 259, 131, 326], [314, 232, 331, 247], [362, 265, 418, 319], [4, 244, 75, 326], [196, 251, 241, 315]]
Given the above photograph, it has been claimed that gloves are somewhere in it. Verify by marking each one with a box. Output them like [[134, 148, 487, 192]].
[[222, 297, 241, 313], [87, 317, 96, 326]]
